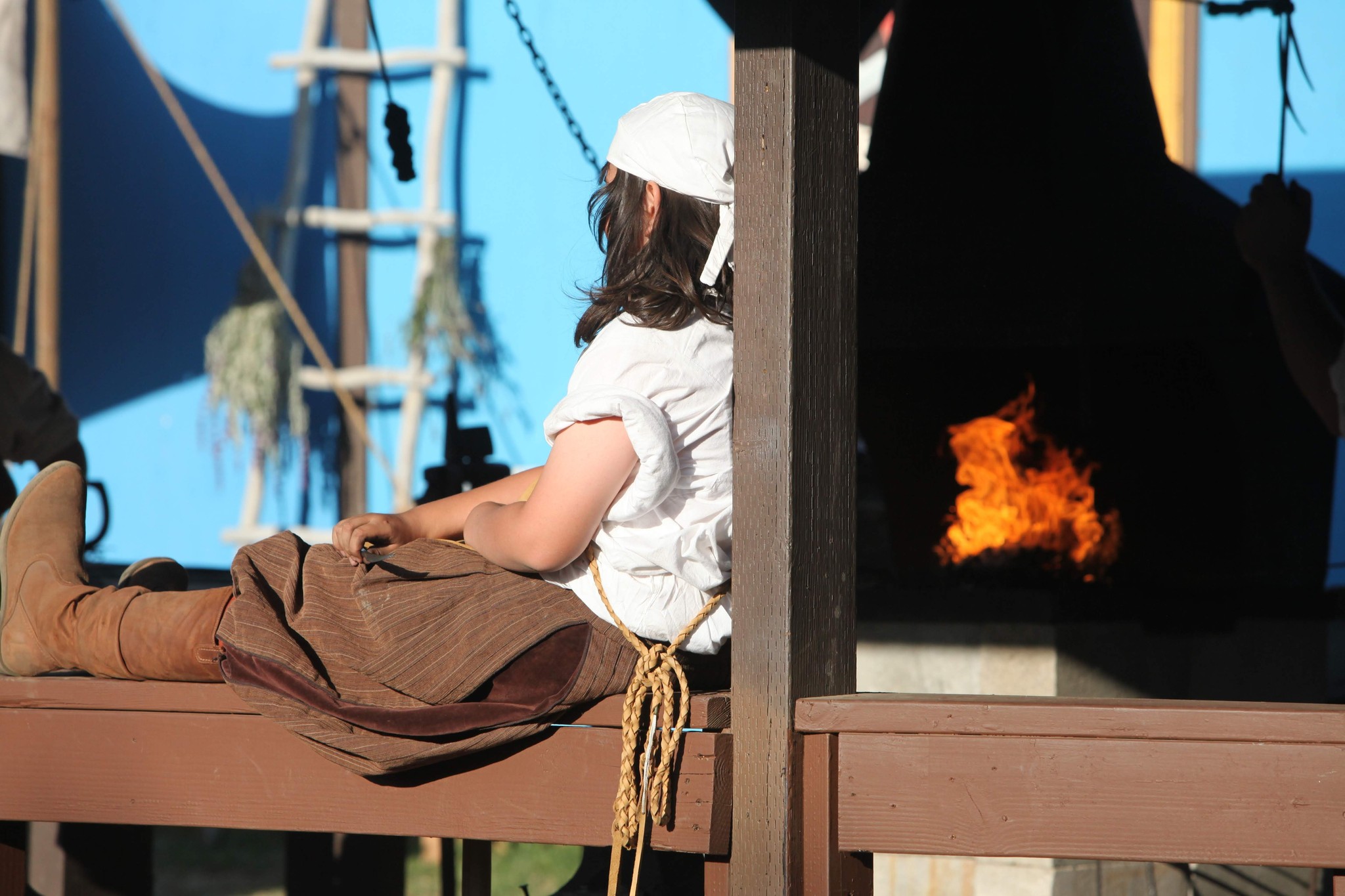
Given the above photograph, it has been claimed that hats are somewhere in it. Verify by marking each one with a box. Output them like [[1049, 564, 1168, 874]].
[[605, 91, 734, 286]]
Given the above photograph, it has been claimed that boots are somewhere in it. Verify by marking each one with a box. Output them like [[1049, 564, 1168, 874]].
[[0, 459, 233, 681]]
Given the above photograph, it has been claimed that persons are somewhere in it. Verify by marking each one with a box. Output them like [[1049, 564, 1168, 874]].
[[1, 89, 735, 779]]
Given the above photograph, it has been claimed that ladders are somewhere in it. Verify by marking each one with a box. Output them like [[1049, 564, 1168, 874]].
[[221, 0, 469, 552]]
